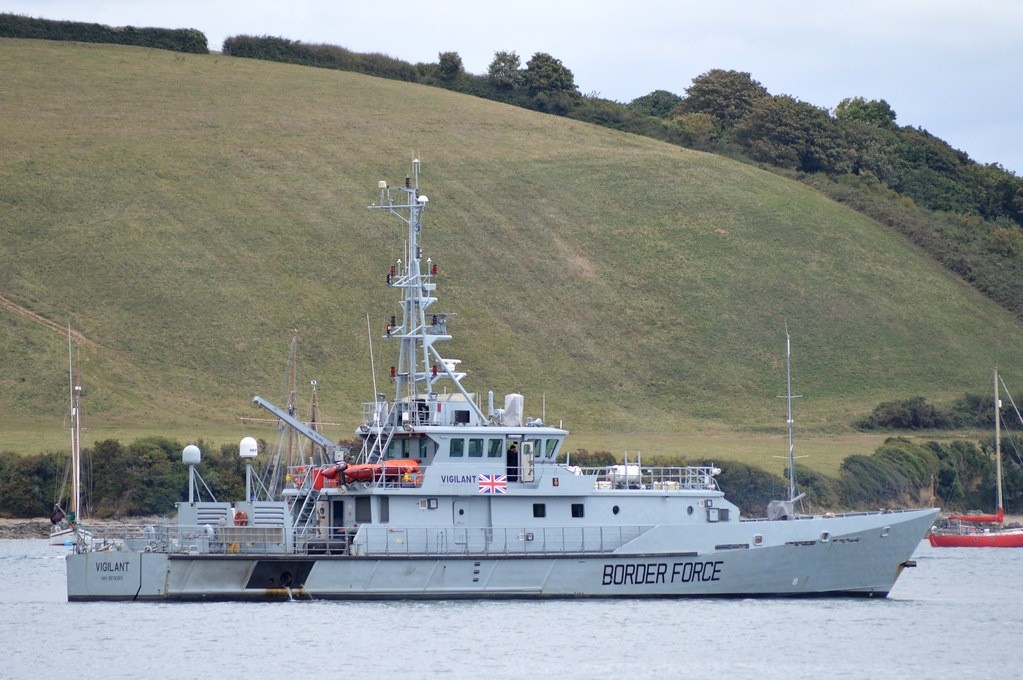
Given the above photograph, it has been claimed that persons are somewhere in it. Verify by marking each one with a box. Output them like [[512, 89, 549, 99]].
[[507, 445, 518, 481]]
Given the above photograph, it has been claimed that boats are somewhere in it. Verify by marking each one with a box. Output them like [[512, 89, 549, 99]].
[[67, 160, 941, 603]]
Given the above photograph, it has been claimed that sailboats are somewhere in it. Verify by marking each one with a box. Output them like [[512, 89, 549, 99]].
[[49, 323, 94, 545], [927, 367, 1023, 547]]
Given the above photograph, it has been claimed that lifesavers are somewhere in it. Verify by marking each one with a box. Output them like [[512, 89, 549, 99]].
[[230, 541, 240, 553], [294, 467, 306, 488], [234, 511, 247, 525], [405, 467, 421, 486]]
